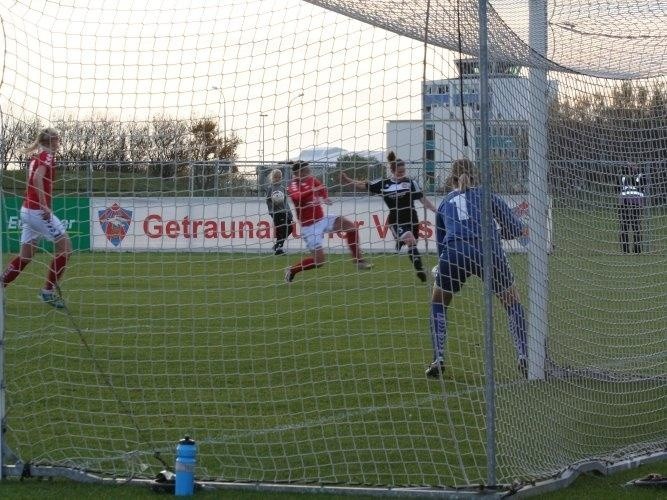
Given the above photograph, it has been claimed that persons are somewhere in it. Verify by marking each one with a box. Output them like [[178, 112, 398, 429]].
[[3, 127, 73, 308], [284, 159, 375, 283], [265, 169, 295, 256], [614, 159, 647, 254], [342, 150, 437, 283], [425, 158, 531, 379]]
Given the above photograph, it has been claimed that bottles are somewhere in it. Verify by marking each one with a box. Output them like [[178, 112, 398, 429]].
[[173, 436, 198, 495]]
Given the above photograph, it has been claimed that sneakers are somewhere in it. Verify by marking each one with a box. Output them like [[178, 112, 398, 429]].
[[272, 244, 287, 256], [284, 266, 294, 283], [38, 291, 64, 308], [516, 355, 527, 377], [357, 263, 374, 268], [425, 360, 444, 378]]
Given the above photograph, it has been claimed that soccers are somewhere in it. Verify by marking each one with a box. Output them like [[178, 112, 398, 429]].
[[432, 264, 440, 279], [271, 190, 286, 204]]
